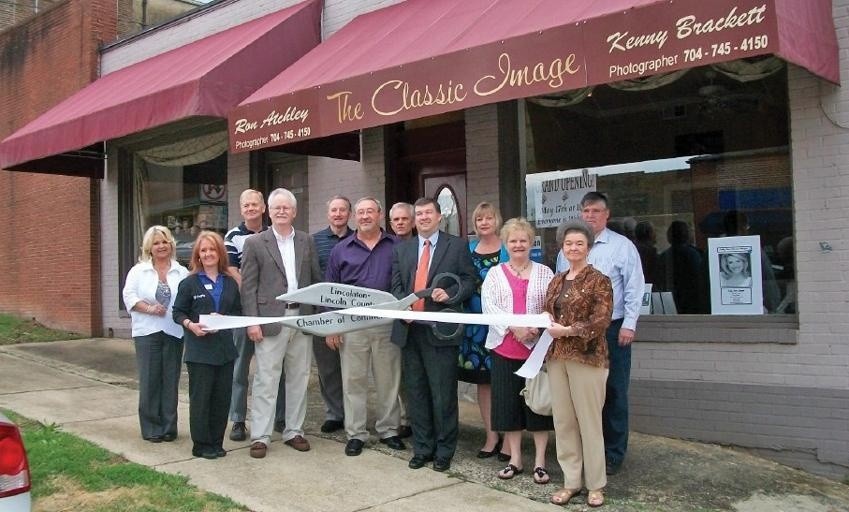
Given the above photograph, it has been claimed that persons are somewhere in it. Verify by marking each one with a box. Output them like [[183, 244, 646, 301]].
[[390, 197, 477, 472], [608, 207, 795, 315], [324, 196, 406, 457], [171, 231, 241, 460], [241, 187, 326, 458], [378, 200, 416, 444], [170, 213, 209, 237], [555, 191, 645, 475], [309, 194, 355, 434], [480, 217, 557, 485], [456, 201, 511, 461], [538, 220, 614, 507], [121, 224, 192, 443], [223, 189, 286, 441]]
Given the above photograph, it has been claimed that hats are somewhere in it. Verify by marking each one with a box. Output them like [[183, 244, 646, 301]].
[[557, 220, 594, 248]]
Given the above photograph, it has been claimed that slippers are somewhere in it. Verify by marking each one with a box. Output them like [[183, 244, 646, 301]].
[[532, 466, 550, 484], [498, 464, 524, 479]]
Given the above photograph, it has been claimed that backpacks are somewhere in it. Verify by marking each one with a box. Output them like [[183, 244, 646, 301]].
[[521, 363, 551, 416]]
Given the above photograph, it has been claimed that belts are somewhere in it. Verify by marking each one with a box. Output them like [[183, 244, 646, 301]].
[[286, 303, 299, 309]]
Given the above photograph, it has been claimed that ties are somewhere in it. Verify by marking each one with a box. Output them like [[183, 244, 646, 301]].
[[412, 240, 430, 311]]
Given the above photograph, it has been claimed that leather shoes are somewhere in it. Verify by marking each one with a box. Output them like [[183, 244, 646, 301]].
[[478, 435, 510, 460], [607, 458, 620, 473], [250, 442, 266, 458], [275, 422, 284, 432], [409, 454, 449, 472], [230, 422, 245, 440], [321, 420, 343, 432], [192, 446, 225, 458], [380, 426, 411, 449], [345, 439, 364, 456], [285, 434, 309, 451]]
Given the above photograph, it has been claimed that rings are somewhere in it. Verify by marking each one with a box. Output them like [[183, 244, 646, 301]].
[[157, 309, 160, 313], [558, 336, 561, 340], [251, 336, 253, 341]]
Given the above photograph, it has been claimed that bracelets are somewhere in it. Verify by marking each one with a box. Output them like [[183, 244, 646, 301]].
[[144, 304, 151, 313], [565, 326, 570, 339], [184, 319, 191, 329]]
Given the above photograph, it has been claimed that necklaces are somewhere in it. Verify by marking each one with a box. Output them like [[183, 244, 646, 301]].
[[509, 261, 531, 279]]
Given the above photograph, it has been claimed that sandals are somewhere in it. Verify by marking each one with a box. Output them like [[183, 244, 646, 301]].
[[550, 488, 581, 505], [588, 490, 604, 506]]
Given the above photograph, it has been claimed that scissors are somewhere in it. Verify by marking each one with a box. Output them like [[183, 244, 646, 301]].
[[276, 272, 464, 340]]
